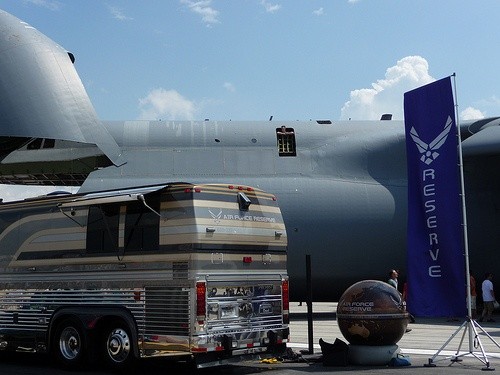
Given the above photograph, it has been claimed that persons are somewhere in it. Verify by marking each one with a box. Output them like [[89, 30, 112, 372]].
[[465, 270, 476, 322], [479, 273, 496, 322], [387, 269, 415, 332]]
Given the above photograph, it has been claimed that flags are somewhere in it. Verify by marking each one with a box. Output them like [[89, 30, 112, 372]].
[[402, 77, 468, 317]]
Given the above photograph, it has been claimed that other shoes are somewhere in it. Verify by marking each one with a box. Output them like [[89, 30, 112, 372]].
[[406, 329, 412, 332]]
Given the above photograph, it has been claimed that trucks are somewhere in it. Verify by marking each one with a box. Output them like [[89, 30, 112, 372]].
[[0, 182, 290, 375]]
